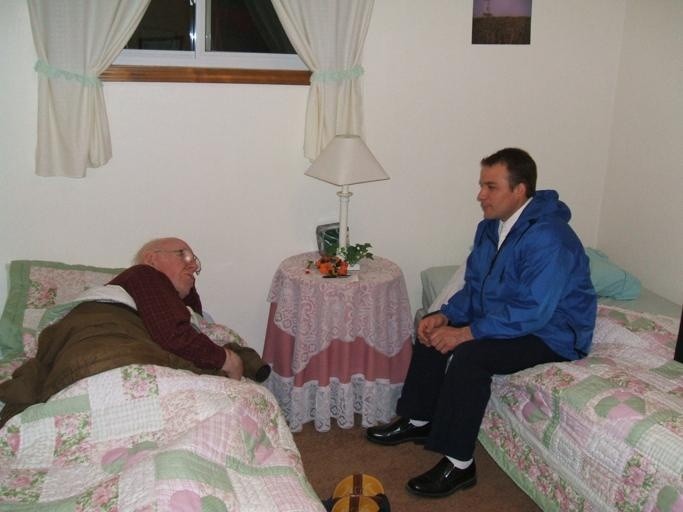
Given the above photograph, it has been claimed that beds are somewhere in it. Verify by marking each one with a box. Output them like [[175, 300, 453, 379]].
[[0, 259, 326, 511], [413, 247, 683, 512]]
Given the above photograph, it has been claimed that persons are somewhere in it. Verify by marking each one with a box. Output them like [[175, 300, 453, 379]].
[[105, 236, 244, 382], [361, 148, 599, 499]]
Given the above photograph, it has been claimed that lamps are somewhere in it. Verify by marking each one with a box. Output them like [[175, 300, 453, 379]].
[[305, 134, 390, 270]]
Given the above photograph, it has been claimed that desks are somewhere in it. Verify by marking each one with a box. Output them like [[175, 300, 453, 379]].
[[262, 250, 413, 434]]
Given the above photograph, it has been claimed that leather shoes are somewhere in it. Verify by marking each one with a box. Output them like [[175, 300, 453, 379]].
[[365, 415, 431, 446], [404, 456, 476, 499]]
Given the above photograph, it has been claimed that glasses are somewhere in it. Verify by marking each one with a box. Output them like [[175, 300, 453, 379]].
[[154, 249, 201, 276]]
